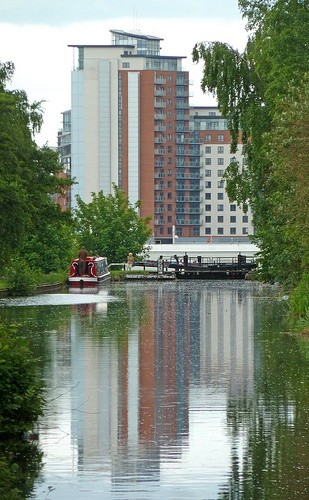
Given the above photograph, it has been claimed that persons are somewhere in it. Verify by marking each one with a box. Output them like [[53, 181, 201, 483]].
[[183, 252, 189, 266], [237, 252, 245, 263], [174, 254, 180, 262], [125, 252, 134, 271], [78, 245, 87, 276]]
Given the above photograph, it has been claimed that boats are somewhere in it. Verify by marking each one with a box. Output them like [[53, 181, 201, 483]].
[[67, 246, 111, 285]]
